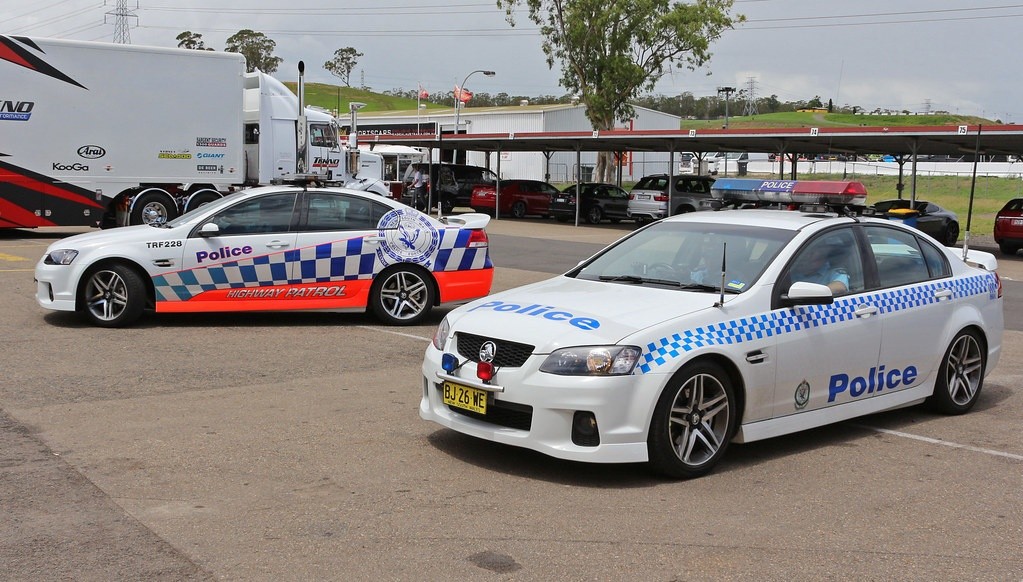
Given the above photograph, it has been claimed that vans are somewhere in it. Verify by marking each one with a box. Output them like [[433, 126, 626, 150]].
[[403, 163, 503, 214]]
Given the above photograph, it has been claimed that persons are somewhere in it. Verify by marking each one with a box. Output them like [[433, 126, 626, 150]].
[[791, 237, 849, 296], [406, 167, 431, 214]]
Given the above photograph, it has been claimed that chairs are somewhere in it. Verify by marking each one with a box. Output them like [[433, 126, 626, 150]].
[[833, 235, 859, 291], [653, 185, 663, 189], [582, 188, 591, 194], [722, 233, 760, 286], [879, 258, 919, 286], [676, 184, 686, 191], [350, 206, 369, 228], [273, 203, 291, 231], [693, 183, 702, 191]]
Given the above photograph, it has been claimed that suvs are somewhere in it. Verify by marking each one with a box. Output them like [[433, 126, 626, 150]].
[[626, 173, 718, 223]]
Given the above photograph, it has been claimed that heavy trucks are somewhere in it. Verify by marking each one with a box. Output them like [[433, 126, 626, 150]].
[[0, 33, 394, 238]]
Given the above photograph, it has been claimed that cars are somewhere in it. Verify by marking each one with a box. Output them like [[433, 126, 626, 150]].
[[862, 199, 960, 247], [679, 152, 895, 174], [548, 182, 644, 225], [32, 172, 497, 326], [470, 179, 562, 220], [994, 197, 1023, 256], [413, 176, 1007, 481]]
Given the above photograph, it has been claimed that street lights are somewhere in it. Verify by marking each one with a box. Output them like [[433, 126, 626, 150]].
[[456, 69, 496, 132]]
